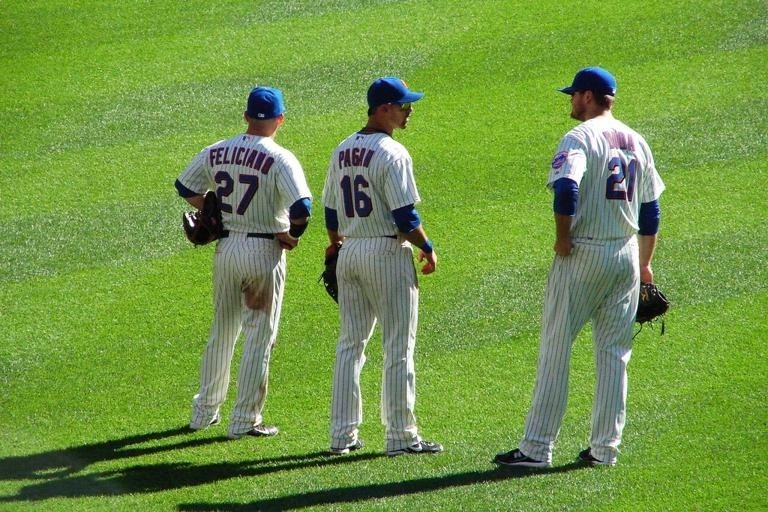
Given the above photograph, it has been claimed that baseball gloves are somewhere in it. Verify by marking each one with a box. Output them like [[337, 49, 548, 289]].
[[183, 190, 225, 246], [635, 280, 669, 321], [325, 240, 342, 302]]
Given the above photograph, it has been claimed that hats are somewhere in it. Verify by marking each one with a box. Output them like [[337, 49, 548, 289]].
[[556, 68, 617, 95], [366, 76, 424, 108], [248, 88, 284, 119]]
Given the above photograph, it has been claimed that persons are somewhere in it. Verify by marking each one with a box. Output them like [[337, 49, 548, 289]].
[[494, 66, 668, 467], [176, 85, 313, 437], [322, 79, 442, 454]]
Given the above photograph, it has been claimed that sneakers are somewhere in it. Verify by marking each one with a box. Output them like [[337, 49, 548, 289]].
[[578, 447, 617, 466], [495, 449, 552, 468], [330, 439, 362, 456], [234, 424, 278, 437], [386, 441, 443, 456]]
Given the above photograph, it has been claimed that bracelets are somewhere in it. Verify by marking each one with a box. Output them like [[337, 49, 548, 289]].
[[419, 240, 434, 254], [287, 222, 309, 240]]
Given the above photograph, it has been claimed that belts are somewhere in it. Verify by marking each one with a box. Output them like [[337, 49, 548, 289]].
[[384, 235, 399, 239], [216, 229, 274, 240]]
